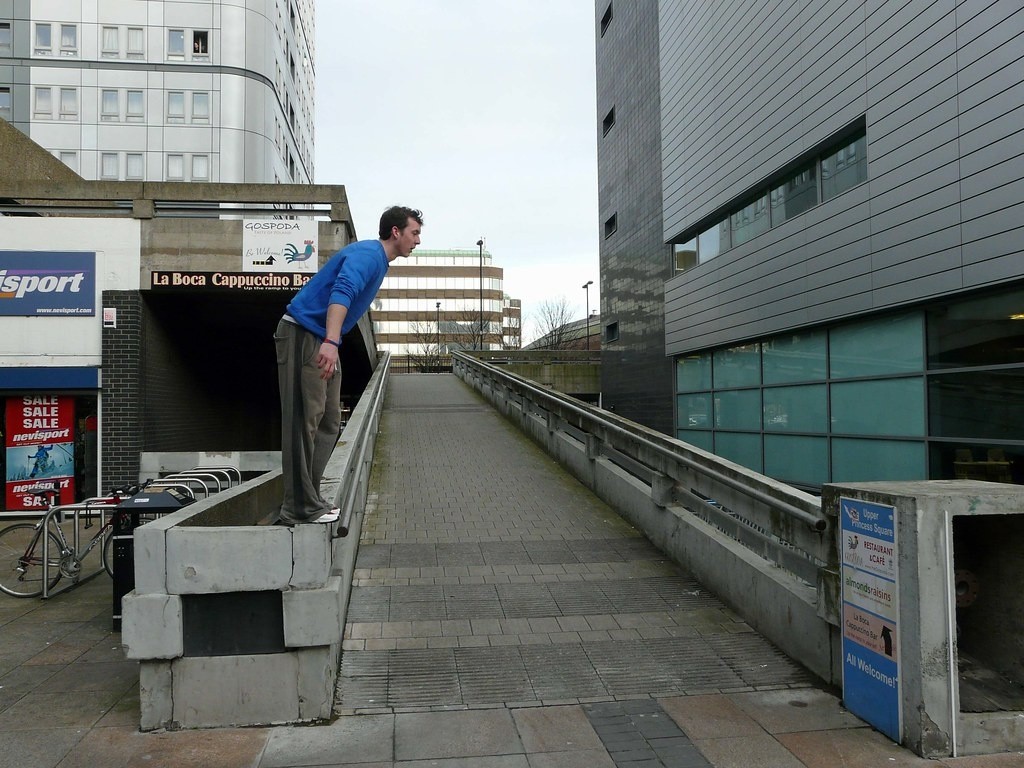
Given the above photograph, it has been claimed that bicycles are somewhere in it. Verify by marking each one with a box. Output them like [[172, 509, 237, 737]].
[[1, 478, 155, 599]]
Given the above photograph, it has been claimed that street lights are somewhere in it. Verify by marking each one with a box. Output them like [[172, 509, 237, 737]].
[[436, 303, 441, 359], [476, 240, 483, 350], [582, 281, 593, 350]]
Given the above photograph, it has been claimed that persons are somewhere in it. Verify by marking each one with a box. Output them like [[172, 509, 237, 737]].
[[27, 444, 57, 479], [274, 205, 422, 523]]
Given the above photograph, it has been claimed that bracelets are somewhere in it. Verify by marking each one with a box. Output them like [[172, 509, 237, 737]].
[[323, 338, 339, 348]]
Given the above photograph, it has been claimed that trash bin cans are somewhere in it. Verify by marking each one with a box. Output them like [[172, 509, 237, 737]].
[[111, 486, 197, 632]]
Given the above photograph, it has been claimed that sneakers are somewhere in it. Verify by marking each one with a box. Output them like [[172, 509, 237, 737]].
[[329, 507, 341, 515], [309, 513, 339, 524]]
